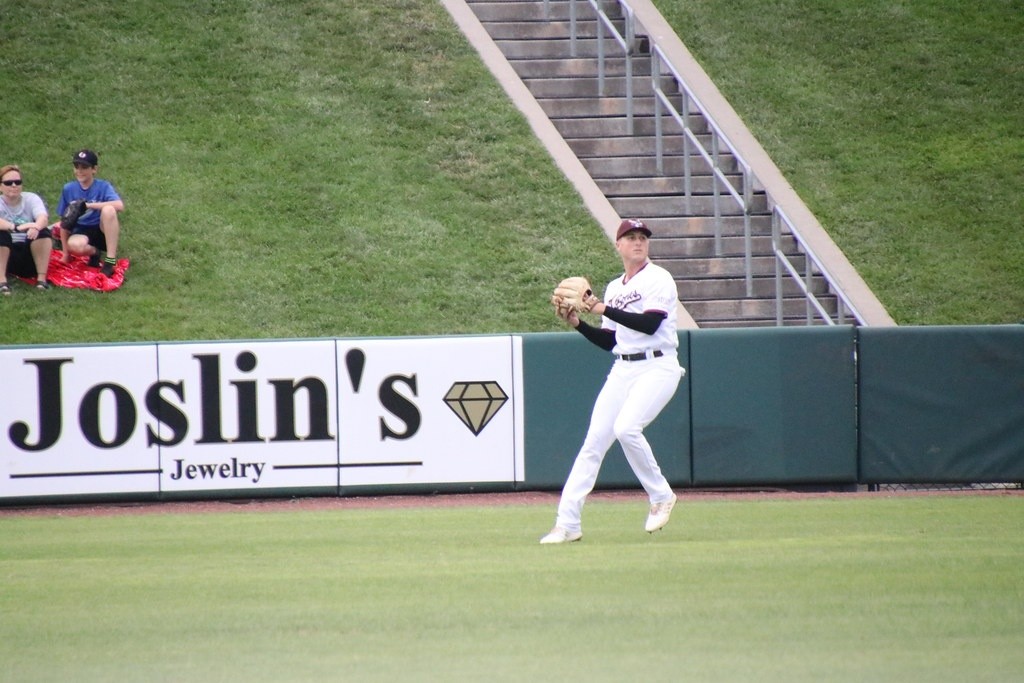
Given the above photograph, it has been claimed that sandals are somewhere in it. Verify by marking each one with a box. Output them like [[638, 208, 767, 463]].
[[36, 281, 49, 289], [0, 283, 11, 292]]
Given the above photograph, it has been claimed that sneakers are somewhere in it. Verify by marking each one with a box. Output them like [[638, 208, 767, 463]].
[[88, 249, 101, 267], [645, 494, 677, 534], [100, 262, 116, 277], [540, 528, 582, 544]]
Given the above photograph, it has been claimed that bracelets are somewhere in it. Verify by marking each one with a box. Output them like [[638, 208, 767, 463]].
[[15, 225, 19, 232]]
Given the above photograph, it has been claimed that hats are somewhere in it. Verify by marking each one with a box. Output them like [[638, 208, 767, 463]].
[[68, 150, 97, 166], [616, 219, 652, 241]]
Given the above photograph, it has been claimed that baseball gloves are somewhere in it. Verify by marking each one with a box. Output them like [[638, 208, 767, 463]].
[[551, 276, 601, 314], [59, 198, 88, 234]]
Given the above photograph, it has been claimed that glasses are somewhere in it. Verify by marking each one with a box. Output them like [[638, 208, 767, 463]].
[[0, 180, 21, 186]]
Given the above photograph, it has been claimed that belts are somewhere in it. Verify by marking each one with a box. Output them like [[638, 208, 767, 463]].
[[617, 350, 663, 360]]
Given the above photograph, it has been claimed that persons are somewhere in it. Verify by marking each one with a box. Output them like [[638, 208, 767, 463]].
[[0, 165, 53, 296], [538, 219, 682, 546], [56, 149, 124, 278]]
